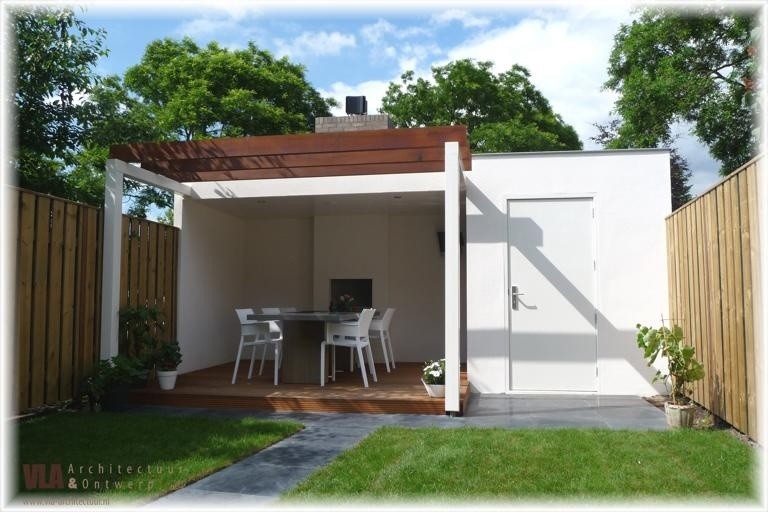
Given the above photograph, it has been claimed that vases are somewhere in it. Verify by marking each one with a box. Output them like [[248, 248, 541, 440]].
[[422, 378, 444, 397]]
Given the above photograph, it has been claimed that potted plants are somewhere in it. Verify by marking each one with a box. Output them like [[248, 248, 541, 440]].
[[152, 339, 182, 390], [637, 322, 704, 431]]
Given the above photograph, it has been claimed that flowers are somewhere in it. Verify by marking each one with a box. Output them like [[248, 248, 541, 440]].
[[421, 357, 445, 385]]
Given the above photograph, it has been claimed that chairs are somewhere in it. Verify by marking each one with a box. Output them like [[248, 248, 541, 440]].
[[229, 307, 395, 391]]
